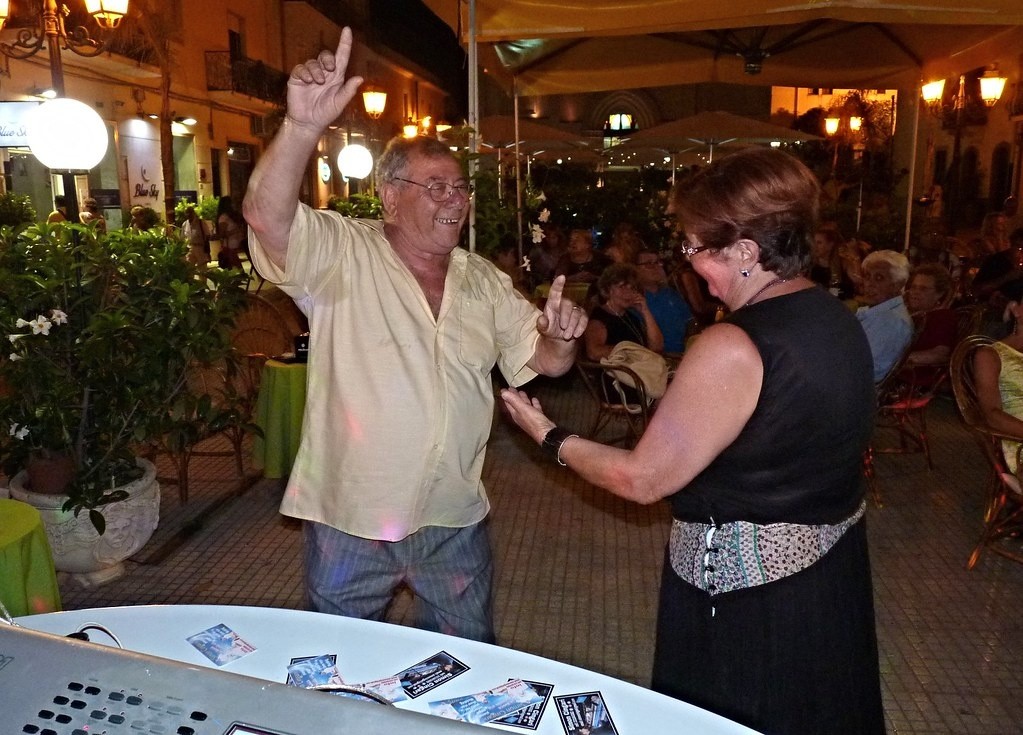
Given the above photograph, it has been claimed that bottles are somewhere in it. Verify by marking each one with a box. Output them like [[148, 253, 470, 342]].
[[829, 273, 839, 297]]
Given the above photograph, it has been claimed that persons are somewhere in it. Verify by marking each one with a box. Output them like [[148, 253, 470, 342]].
[[502, 146, 886, 735], [242, 27, 588, 646], [47, 195, 246, 284], [490, 216, 951, 405], [900, 211, 1023, 474]]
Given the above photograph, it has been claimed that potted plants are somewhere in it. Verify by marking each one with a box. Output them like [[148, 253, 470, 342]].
[[0, 222, 237, 585]]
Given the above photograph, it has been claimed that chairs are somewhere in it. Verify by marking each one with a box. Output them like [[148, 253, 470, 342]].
[[878, 308, 983, 471], [871, 303, 926, 395], [576, 359, 669, 449], [950, 334, 1022, 572]]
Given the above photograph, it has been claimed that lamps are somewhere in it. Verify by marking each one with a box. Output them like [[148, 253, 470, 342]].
[[137, 110, 158, 119], [176, 116, 198, 126], [34, 88, 57, 99]]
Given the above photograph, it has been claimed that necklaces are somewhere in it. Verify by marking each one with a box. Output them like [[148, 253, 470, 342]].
[[606, 301, 643, 344]]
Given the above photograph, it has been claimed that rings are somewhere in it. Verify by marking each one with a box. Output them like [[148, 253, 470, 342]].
[[572, 306, 581, 312]]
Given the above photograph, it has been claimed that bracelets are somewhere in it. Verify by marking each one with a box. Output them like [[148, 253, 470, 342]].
[[542, 427, 579, 466], [742, 271, 800, 306]]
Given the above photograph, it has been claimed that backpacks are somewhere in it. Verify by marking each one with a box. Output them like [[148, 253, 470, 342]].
[[600, 341, 670, 415]]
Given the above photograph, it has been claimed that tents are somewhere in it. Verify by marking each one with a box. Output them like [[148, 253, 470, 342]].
[[434, 1, 1023, 280]]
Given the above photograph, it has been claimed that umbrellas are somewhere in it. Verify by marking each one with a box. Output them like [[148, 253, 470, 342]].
[[436, 109, 825, 206]]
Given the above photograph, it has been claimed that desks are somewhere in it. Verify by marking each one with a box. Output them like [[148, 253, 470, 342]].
[[535, 282, 592, 306], [253, 357, 309, 478], [0, 497, 62, 618], [2, 605, 765, 735]]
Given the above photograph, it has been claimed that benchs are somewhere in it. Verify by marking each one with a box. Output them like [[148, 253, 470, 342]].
[[139, 408, 249, 507]]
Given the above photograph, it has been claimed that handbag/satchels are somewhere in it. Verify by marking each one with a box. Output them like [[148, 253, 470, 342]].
[[218, 247, 232, 271], [203, 239, 210, 253]]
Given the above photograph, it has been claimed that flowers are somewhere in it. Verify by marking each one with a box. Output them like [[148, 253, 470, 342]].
[[518, 191, 550, 273]]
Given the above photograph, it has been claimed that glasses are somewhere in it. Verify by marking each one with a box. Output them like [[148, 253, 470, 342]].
[[639, 259, 663, 269], [680, 240, 762, 262], [393, 177, 474, 202]]
